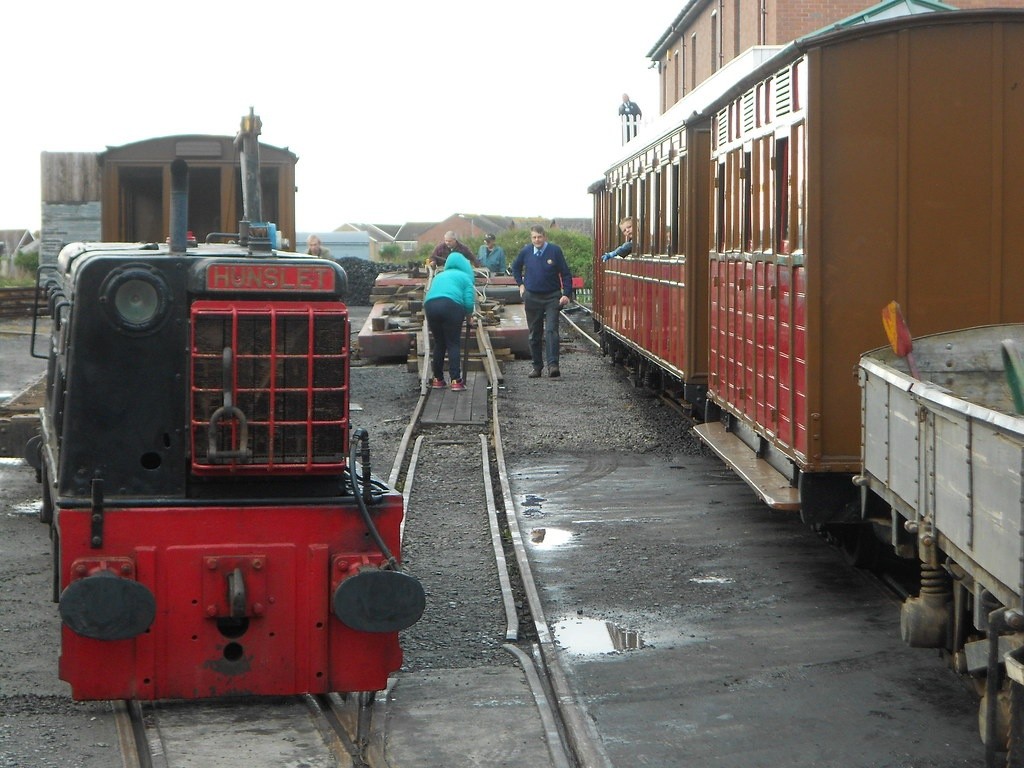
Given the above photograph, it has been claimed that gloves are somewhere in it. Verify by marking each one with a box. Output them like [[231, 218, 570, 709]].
[[601, 251, 618, 263]]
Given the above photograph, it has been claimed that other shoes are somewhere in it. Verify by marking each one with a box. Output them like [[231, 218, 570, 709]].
[[548, 366, 560, 377], [528, 368, 541, 377]]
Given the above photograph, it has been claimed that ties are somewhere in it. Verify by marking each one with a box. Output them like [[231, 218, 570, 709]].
[[537, 251, 541, 256]]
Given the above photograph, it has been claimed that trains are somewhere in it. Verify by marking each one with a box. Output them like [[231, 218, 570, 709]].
[[23, 105, 428, 702], [589, 1, 1024, 767]]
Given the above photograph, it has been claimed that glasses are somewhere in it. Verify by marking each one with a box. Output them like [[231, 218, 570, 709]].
[[486, 240, 492, 244]]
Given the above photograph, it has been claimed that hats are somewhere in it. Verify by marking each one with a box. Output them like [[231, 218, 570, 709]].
[[484, 234, 496, 240]]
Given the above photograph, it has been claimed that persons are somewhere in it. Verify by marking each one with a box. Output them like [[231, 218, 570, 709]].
[[306, 234, 333, 260], [618, 93, 642, 142], [424, 252, 475, 391], [477, 233, 506, 273], [601, 216, 632, 262], [432, 231, 480, 268], [512, 225, 572, 378]]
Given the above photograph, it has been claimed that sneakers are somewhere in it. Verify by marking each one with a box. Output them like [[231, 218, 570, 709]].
[[432, 378, 446, 389], [451, 378, 467, 391]]
